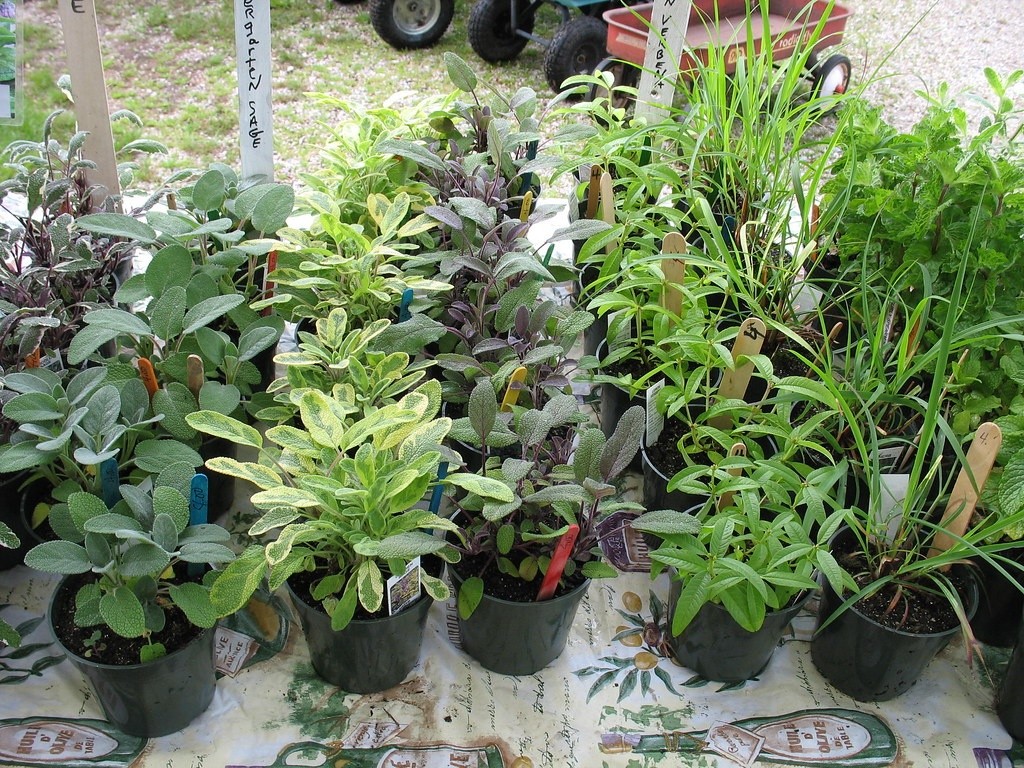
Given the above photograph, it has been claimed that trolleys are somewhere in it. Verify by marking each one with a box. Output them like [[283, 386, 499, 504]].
[[586, 1, 852, 126], [467, 0, 646, 102]]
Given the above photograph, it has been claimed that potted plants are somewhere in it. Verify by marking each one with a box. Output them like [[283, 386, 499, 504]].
[[0, 1, 1024, 744]]
